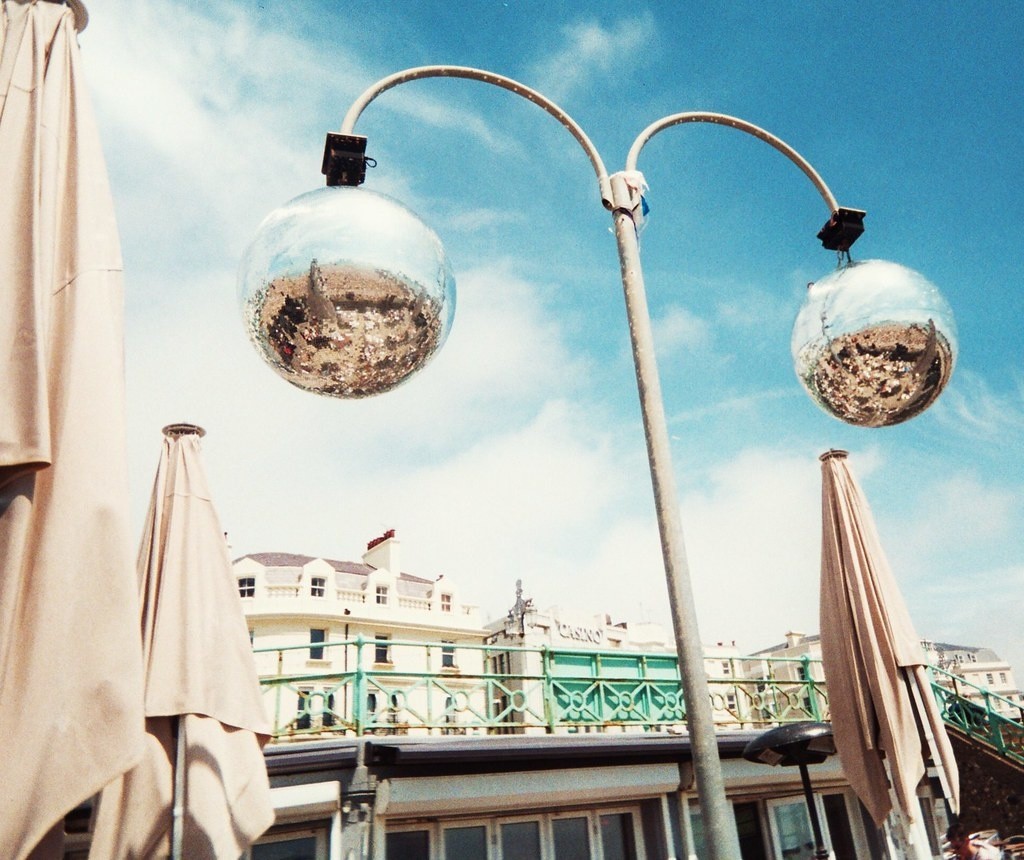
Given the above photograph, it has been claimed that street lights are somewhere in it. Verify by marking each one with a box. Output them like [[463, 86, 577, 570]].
[[237, 64, 958, 860]]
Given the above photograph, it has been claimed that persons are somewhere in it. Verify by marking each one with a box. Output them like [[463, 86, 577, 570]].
[[946, 823, 1004, 860]]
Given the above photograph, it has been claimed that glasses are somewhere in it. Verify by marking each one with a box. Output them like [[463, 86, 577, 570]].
[[949, 843, 964, 850]]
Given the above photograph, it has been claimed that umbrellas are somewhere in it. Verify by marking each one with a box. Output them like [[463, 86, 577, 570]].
[[0, 0, 147, 860], [818, 450, 960, 849], [81, 423, 280, 860]]
